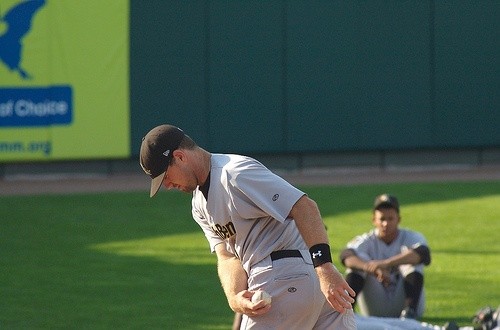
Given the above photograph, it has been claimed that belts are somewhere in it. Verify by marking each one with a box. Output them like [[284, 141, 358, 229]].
[[270, 250, 302, 261]]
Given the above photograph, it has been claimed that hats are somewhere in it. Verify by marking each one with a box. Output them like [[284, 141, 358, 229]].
[[374, 194, 398, 211], [139, 125, 184, 198]]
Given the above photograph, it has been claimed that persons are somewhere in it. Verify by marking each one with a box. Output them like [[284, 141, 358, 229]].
[[139, 124, 357, 330], [340, 195, 432, 321]]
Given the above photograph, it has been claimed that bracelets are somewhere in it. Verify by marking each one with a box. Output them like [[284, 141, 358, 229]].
[[309, 244, 332, 268]]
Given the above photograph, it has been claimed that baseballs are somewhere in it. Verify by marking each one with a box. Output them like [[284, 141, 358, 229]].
[[250, 290, 272, 304]]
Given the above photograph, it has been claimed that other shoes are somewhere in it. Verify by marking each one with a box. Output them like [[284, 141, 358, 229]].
[[445, 321, 459, 330], [472, 303, 500, 330]]
